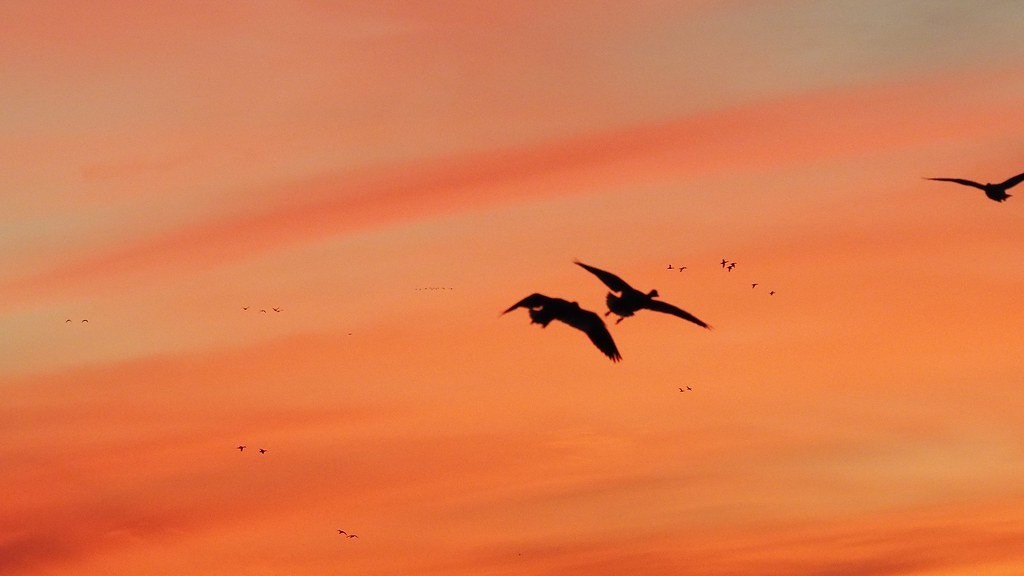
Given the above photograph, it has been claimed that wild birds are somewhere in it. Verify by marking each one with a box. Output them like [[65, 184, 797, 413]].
[[65, 318, 89, 323], [337, 529, 358, 539], [752, 283, 776, 296], [922, 173, 1024, 203], [243, 305, 285, 316], [678, 386, 693, 393], [237, 445, 267, 455], [500, 261, 714, 362], [720, 259, 737, 273]]
